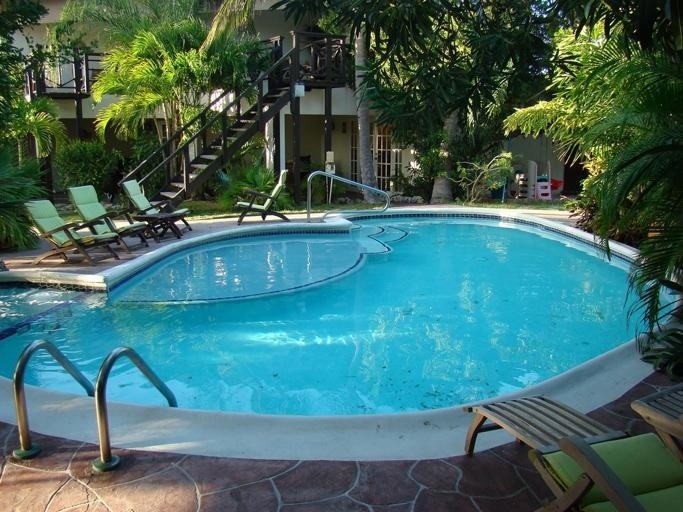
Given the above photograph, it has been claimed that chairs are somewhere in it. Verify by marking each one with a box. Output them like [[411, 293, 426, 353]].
[[22, 168, 290, 268], [462, 379, 683, 512]]
